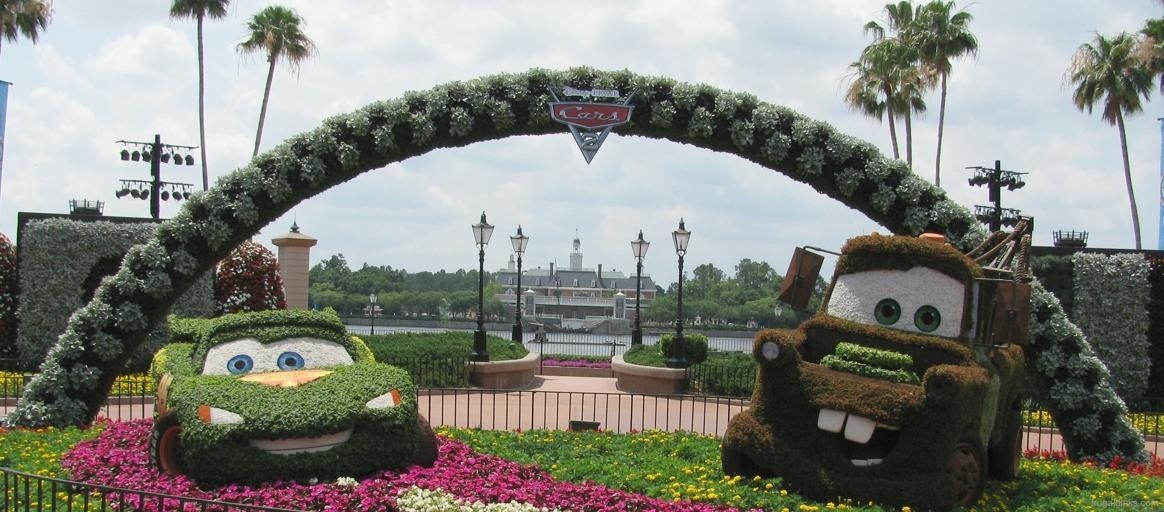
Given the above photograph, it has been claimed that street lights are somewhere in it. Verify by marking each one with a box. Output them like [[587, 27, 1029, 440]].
[[965, 158, 1035, 237], [465, 211, 494, 360], [671, 217, 694, 336], [114, 134, 197, 217], [627, 228, 649, 344], [365, 289, 380, 336], [507, 223, 528, 344]]
